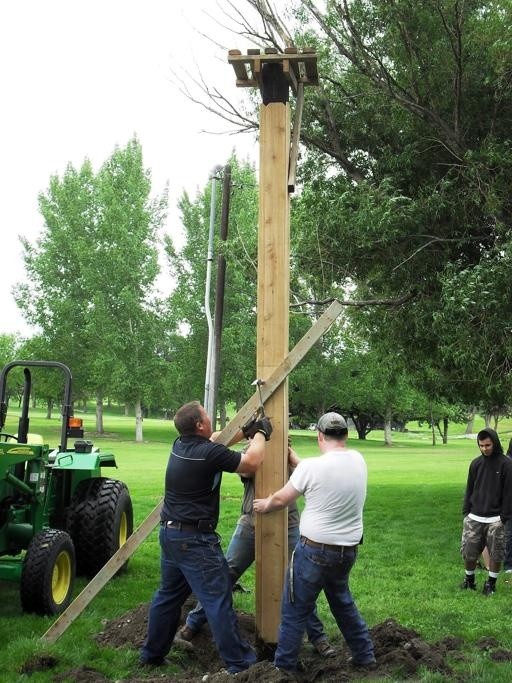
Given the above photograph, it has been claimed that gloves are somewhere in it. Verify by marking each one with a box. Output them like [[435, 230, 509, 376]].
[[241, 417, 274, 440]]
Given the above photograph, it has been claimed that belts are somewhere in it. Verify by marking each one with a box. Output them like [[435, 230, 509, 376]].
[[162, 521, 197, 531], [300, 536, 359, 552]]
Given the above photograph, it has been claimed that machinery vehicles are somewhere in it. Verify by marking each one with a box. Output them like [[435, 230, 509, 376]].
[[0, 361, 133, 616]]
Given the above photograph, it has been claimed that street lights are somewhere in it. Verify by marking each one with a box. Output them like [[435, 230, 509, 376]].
[[204, 163, 224, 428]]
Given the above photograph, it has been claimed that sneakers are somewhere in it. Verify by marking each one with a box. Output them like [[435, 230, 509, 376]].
[[482, 579, 496, 595], [315, 640, 337, 660], [460, 577, 476, 588], [140, 656, 174, 667], [177, 625, 196, 641]]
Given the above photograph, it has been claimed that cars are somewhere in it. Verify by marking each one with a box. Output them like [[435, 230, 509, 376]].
[[289, 412, 317, 431]]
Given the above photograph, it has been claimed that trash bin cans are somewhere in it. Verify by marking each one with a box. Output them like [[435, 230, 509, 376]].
[[142, 407, 148, 418]]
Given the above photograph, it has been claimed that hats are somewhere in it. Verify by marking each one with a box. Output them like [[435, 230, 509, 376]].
[[317, 411, 348, 435]]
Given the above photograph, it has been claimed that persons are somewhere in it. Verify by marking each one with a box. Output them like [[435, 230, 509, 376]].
[[455, 427, 510, 596], [251, 411, 381, 669], [480, 546, 491, 572], [131, 400, 273, 672], [499, 436, 511, 575], [170, 410, 340, 661]]
